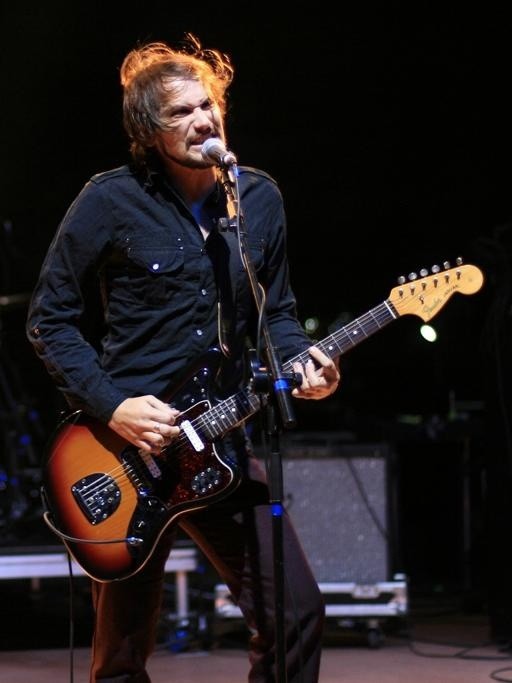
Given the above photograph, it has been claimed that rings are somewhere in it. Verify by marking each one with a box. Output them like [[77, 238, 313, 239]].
[[154, 422, 162, 432]]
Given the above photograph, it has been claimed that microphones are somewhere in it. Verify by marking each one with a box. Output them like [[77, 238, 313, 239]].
[[200, 134, 237, 172]]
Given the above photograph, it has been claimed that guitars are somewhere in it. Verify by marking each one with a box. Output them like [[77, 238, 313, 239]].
[[39, 256, 484, 585]]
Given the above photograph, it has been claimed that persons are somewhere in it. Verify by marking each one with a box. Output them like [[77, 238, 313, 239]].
[[23, 33, 344, 682]]
